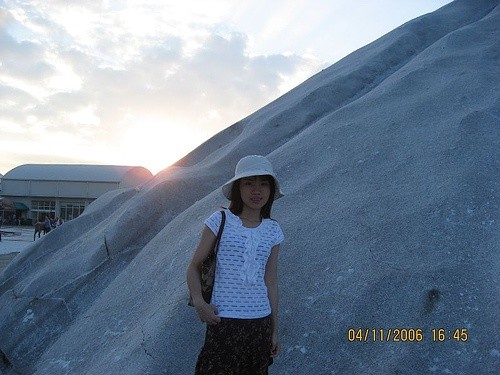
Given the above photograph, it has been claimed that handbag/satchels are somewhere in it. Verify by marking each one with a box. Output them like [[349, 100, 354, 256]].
[[189, 210, 226, 307]]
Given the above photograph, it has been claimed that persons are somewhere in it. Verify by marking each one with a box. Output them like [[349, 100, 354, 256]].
[[187, 155, 285, 375]]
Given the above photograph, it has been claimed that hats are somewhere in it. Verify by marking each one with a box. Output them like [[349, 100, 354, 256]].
[[222, 155, 285, 201]]
